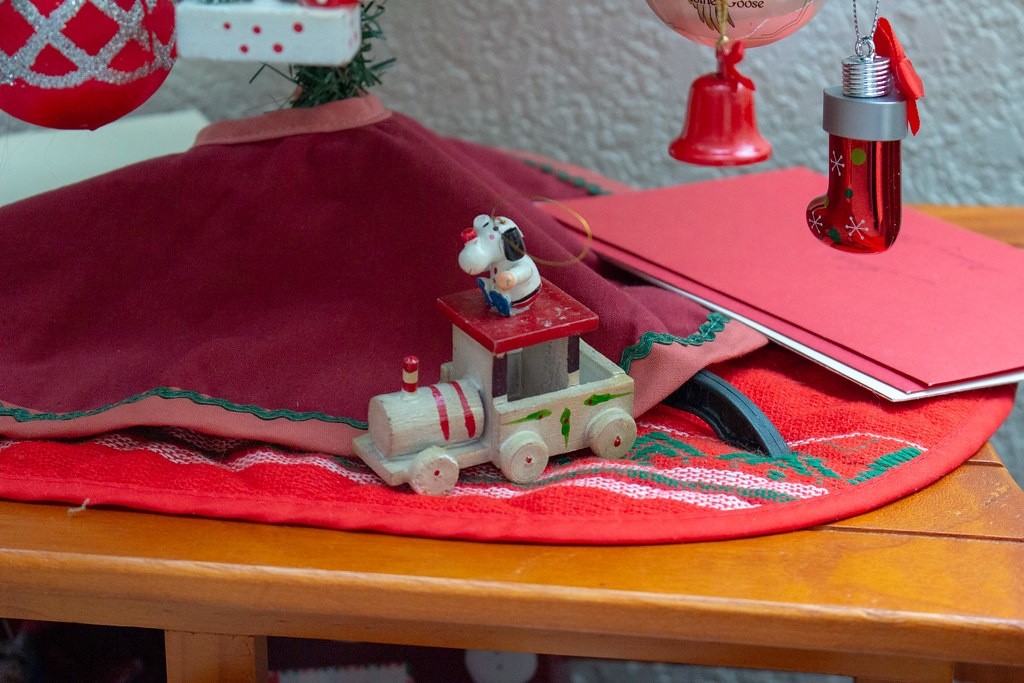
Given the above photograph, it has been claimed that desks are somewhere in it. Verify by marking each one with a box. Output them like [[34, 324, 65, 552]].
[[0, 204, 1024, 682]]
